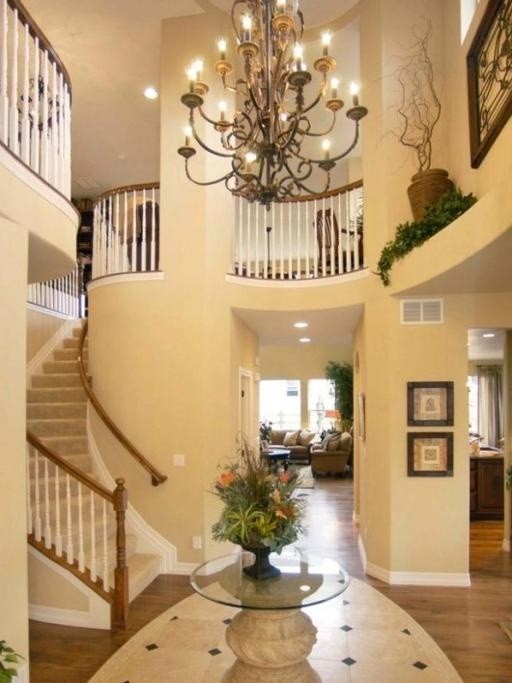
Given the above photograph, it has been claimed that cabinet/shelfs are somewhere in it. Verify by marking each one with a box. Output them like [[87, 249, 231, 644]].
[[469, 456, 505, 521]]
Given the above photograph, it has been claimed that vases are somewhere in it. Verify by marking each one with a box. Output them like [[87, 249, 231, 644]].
[[244, 543, 283, 581], [406, 169, 454, 225]]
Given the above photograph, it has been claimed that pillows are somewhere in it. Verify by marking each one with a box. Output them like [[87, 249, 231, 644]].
[[283, 429, 315, 447], [324, 431, 340, 452]]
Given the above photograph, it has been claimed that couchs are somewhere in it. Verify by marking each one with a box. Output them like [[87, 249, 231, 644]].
[[260, 428, 315, 465]]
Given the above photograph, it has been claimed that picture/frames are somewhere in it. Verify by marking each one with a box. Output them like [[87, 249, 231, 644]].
[[466, 0, 512, 169], [406, 380, 454, 478]]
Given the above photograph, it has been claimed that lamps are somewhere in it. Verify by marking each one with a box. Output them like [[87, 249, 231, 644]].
[[325, 410, 340, 432], [177, 1, 370, 213]]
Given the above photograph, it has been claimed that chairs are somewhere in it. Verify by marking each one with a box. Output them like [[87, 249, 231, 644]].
[[311, 207, 363, 274], [310, 431, 352, 478]]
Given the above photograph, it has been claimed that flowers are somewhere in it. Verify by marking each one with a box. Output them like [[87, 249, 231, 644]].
[[203, 432, 310, 553]]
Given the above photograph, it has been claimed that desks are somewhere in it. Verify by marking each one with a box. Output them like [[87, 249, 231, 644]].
[[188, 550, 350, 683], [263, 448, 292, 474]]
